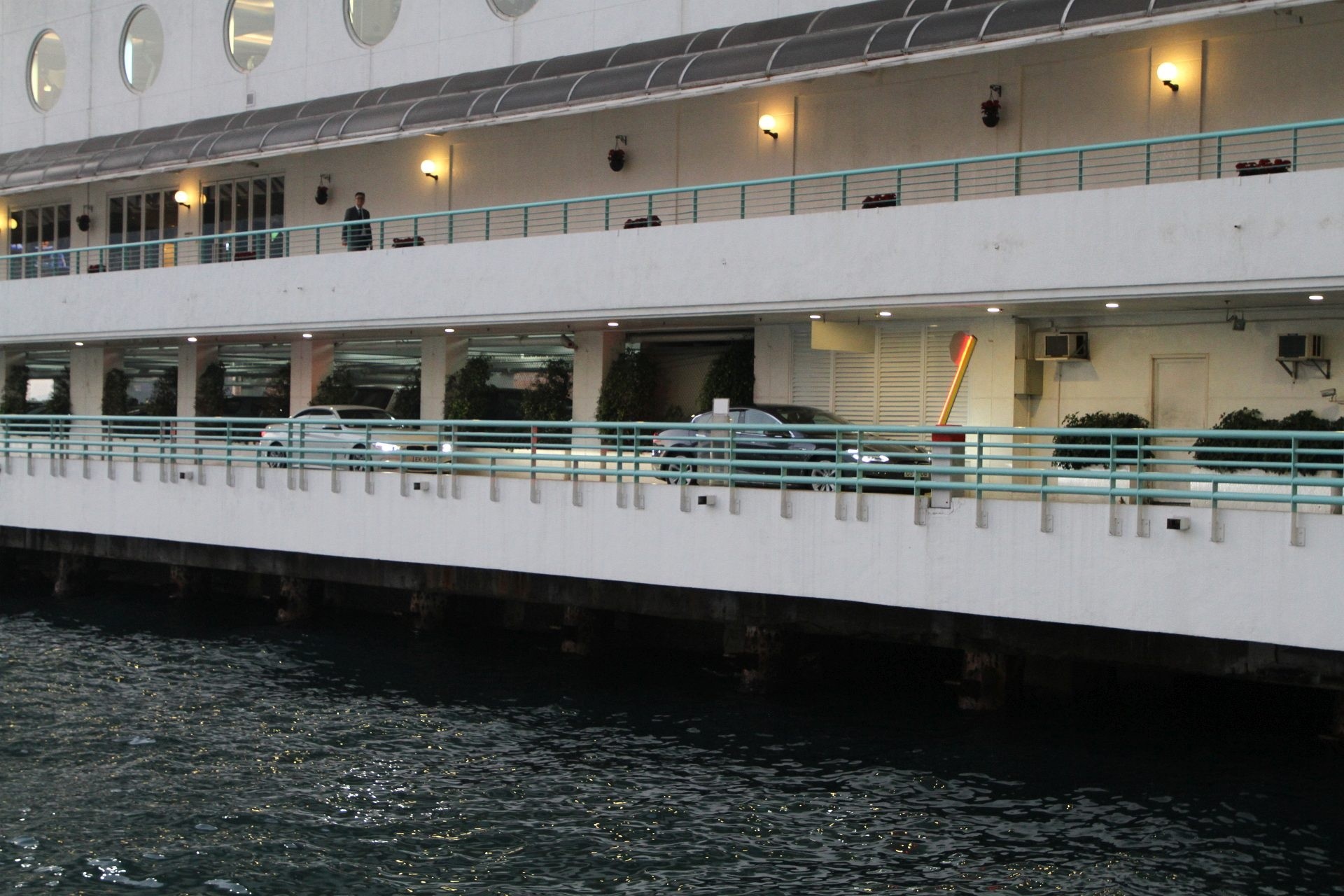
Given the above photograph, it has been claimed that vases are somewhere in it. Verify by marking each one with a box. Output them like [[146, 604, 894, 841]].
[[1239, 168, 1287, 176], [982, 116, 999, 127], [88, 268, 106, 273], [610, 162, 624, 171], [78, 223, 88, 231], [315, 197, 327, 204]]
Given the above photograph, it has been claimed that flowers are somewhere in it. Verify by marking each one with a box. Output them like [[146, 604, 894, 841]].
[[393, 237, 424, 247], [76, 215, 90, 224], [90, 264, 106, 269], [236, 251, 256, 259], [981, 100, 1001, 117], [607, 150, 625, 162], [1236, 159, 1291, 168], [317, 186, 328, 197], [625, 216, 661, 228], [862, 193, 896, 208]]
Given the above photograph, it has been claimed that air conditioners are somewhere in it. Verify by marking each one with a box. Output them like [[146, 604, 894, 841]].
[[1278, 334, 1323, 359], [1044, 333, 1088, 358]]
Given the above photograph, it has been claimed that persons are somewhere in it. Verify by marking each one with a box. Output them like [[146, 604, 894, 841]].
[[342, 192, 373, 252]]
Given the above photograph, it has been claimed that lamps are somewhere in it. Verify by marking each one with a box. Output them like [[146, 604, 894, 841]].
[[420, 160, 438, 179], [174, 191, 190, 208], [759, 114, 778, 139], [1156, 62, 1179, 91]]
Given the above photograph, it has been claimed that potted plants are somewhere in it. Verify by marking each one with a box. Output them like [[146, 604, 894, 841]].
[[1050, 407, 1344, 515], [1, 335, 756, 481]]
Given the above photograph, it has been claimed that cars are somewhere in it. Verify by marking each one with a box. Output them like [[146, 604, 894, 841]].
[[652, 403, 932, 497], [258, 404, 457, 476]]
